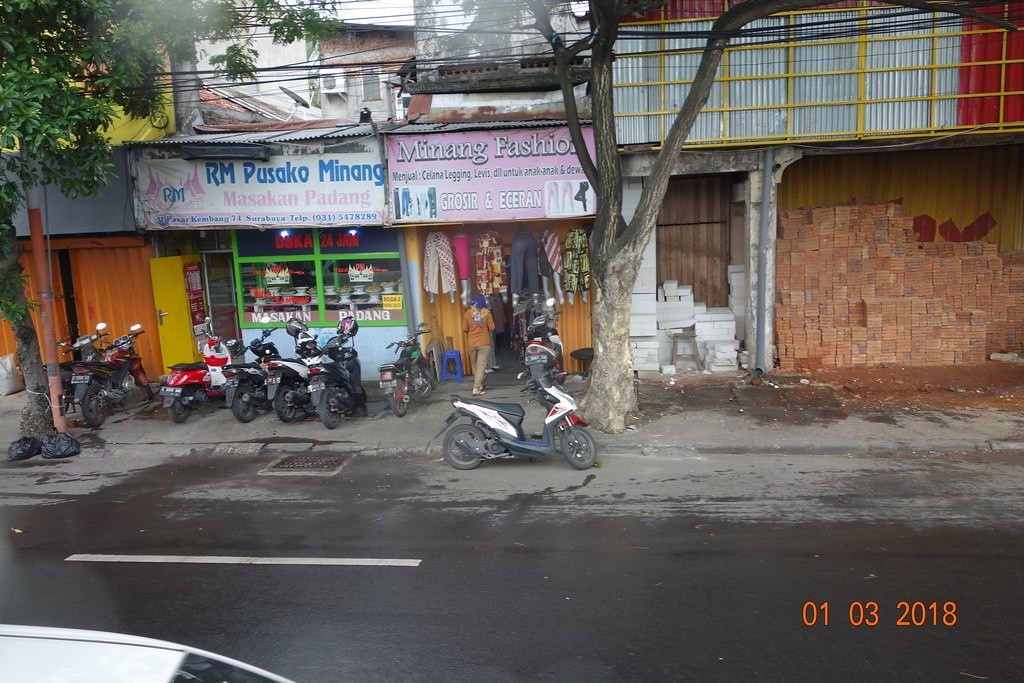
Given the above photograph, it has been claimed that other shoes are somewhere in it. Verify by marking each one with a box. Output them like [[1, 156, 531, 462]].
[[472, 388, 486, 397], [480, 382, 486, 390]]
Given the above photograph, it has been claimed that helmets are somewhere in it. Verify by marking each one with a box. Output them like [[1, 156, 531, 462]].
[[337, 318, 358, 337], [286, 318, 308, 337]]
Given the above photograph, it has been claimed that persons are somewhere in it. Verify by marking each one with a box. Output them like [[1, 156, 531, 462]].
[[461, 293, 496, 396], [422, 230, 458, 305], [510, 228, 565, 309], [473, 228, 509, 306], [450, 231, 472, 308], [563, 226, 590, 306]]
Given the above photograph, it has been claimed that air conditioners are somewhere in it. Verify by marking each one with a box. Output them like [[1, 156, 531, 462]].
[[319, 68, 347, 93]]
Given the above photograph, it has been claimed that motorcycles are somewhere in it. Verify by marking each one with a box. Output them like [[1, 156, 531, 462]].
[[377, 322, 435, 418], [520, 296, 566, 395], [433, 370, 599, 471], [43, 322, 111, 413], [159, 316, 237, 424], [71, 323, 154, 428], [260, 316, 324, 423], [221, 325, 281, 423], [305, 315, 367, 430]]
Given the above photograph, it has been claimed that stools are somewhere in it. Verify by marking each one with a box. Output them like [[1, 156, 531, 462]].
[[672, 332, 704, 369], [570, 347, 594, 377], [440, 349, 464, 384]]
